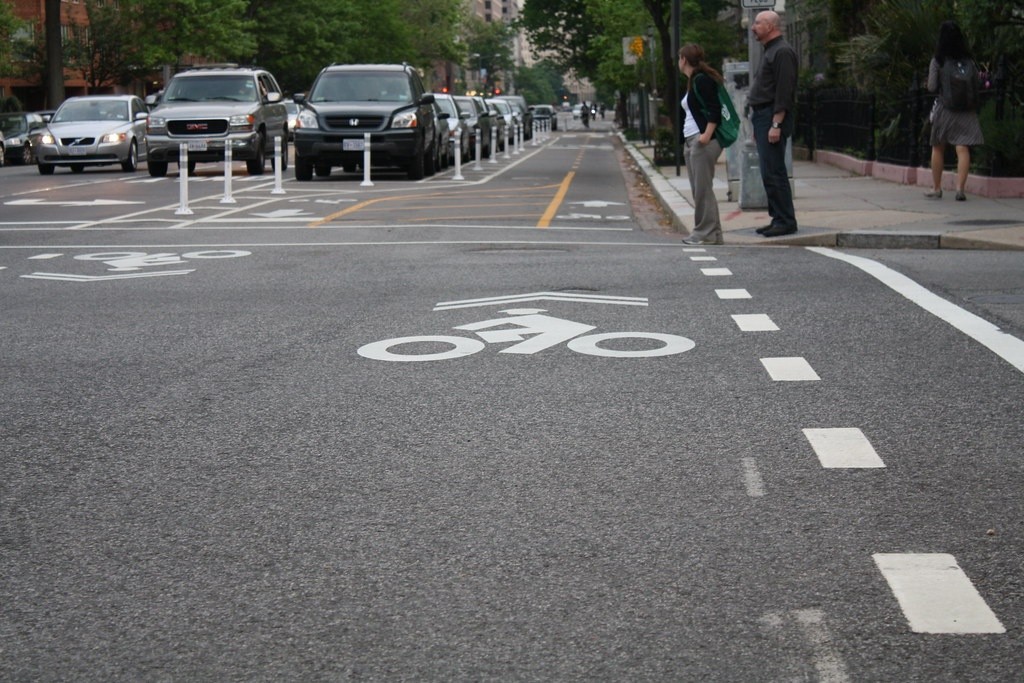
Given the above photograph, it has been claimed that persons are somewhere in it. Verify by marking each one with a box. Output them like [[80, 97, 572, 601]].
[[744, 11, 799, 238], [677, 44, 725, 245], [580, 100, 606, 123], [928, 21, 983, 200]]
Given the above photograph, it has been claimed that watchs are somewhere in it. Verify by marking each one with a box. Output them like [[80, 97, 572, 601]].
[[771, 121, 782, 128]]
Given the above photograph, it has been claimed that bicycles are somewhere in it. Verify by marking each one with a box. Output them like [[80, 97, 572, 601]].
[[581, 113, 588, 127]]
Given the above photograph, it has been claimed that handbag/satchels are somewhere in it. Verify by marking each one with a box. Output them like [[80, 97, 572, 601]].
[[692, 72, 740, 150]]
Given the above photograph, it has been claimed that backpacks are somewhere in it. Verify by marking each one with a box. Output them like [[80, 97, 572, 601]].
[[936, 53, 979, 112]]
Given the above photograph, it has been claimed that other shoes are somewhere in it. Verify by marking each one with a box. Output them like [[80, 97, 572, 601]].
[[682, 234, 717, 245], [923, 188, 942, 200], [763, 224, 797, 237], [956, 192, 966, 201], [756, 222, 798, 234], [717, 239, 724, 245]]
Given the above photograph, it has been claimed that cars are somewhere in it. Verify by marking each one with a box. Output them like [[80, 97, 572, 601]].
[[0, 109, 56, 167], [35, 97, 150, 174], [529, 105, 558, 131], [282, 98, 304, 138], [574, 103, 591, 120], [427, 90, 535, 169]]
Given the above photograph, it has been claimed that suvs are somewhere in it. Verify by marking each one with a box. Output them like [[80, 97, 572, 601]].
[[144, 64, 288, 176], [292, 60, 435, 179]]
